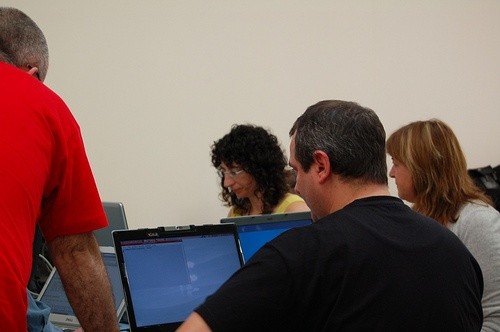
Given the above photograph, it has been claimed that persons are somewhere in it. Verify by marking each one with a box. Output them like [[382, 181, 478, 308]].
[[211, 123, 311, 216], [385, 119, 500, 332], [0, 6, 120, 332], [176, 98, 484, 332]]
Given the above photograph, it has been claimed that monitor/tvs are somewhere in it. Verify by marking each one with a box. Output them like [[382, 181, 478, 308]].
[[35, 245, 127, 332]]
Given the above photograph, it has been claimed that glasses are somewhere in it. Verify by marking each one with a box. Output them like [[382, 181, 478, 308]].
[[217, 167, 244, 178]]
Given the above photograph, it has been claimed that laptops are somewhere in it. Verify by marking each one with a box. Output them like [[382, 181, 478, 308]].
[[91, 202, 130, 246], [113, 222, 246, 332], [220, 211, 314, 262]]
